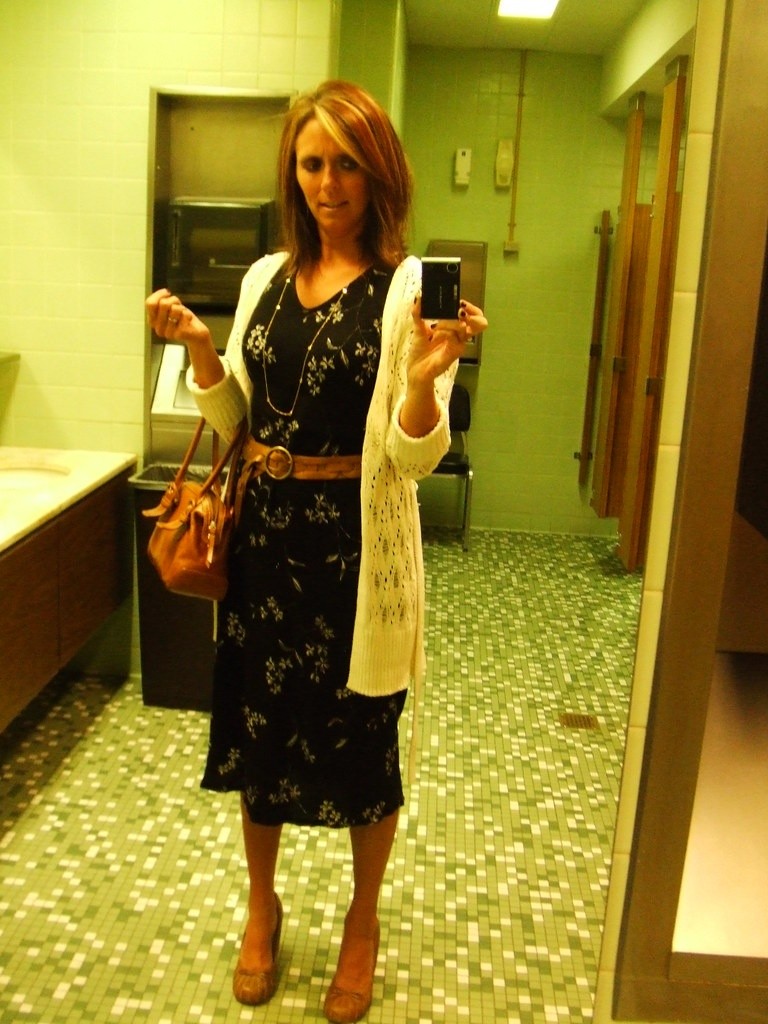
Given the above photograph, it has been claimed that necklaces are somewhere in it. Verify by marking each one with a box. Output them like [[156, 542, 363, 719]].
[[262, 272, 354, 416]]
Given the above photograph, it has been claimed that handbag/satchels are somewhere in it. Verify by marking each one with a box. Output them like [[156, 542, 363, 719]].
[[142, 412, 249, 601]]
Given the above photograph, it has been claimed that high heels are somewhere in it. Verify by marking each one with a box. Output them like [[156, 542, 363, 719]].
[[233, 891, 283, 1005], [323, 919, 380, 1024]]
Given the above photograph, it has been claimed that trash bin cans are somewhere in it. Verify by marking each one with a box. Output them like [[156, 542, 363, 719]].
[[126, 462, 231, 713]]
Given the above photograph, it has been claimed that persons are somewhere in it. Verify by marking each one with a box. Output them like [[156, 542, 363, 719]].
[[148, 82, 490, 1023]]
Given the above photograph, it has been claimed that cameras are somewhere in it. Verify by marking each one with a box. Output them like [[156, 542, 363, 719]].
[[421, 255, 461, 321]]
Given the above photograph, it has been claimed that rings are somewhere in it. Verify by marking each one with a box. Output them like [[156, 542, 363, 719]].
[[167, 316, 179, 325]]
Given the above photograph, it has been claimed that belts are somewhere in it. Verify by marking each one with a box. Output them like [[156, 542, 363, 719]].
[[234, 433, 362, 530]]
